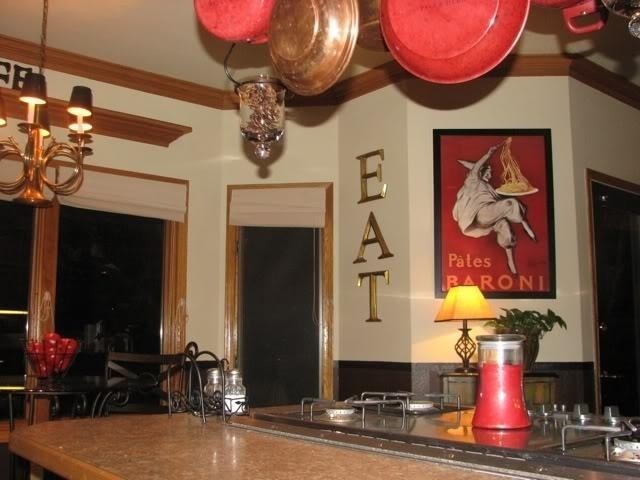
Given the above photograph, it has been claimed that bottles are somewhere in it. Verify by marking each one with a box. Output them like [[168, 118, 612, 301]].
[[203, 367, 248, 415], [471, 334, 532, 429], [474, 429, 532, 452]]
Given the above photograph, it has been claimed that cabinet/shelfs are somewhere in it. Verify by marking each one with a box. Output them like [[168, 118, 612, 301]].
[[439, 373, 559, 407]]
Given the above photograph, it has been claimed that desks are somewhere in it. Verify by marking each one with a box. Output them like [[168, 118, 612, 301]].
[[0, 384, 89, 480]]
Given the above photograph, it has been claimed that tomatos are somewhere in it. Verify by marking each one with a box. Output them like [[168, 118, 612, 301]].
[[27, 331, 78, 375]]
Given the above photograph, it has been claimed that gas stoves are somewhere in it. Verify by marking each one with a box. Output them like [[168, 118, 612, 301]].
[[252, 388, 639, 476]]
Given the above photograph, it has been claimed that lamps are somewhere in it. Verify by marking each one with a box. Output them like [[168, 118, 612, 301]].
[[0, 0, 94, 205], [434, 286, 498, 372]]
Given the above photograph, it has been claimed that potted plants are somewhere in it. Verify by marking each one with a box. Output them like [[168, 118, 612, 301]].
[[483, 307, 567, 372]]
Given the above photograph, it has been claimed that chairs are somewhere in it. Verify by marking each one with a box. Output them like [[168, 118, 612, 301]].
[[103, 341, 230, 416]]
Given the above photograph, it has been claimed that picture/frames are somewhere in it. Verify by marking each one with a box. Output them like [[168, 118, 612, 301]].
[[433, 129, 556, 300]]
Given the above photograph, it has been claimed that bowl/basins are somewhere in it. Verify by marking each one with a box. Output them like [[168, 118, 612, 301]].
[[193, 0, 279, 45]]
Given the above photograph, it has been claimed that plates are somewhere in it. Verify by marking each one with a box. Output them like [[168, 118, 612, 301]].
[[268, 1, 360, 101], [494, 184, 540, 195], [378, 0, 531, 84]]
